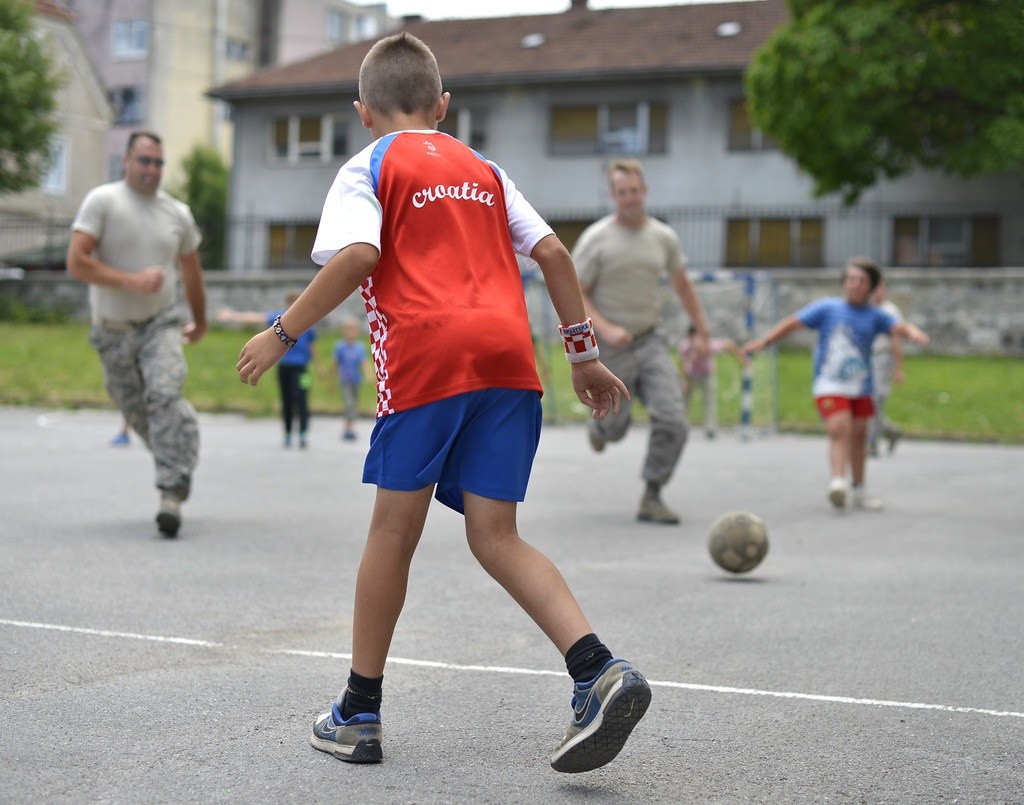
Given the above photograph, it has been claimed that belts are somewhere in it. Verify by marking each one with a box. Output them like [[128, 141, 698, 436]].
[[94, 316, 159, 330]]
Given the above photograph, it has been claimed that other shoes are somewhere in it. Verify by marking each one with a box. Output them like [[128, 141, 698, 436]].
[[156, 505, 180, 536], [829, 482, 850, 510], [590, 429, 604, 451], [344, 433, 356, 439], [638, 501, 679, 522], [110, 433, 128, 446], [854, 496, 887, 511]]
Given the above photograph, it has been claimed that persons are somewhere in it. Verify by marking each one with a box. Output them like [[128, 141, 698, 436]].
[[738, 257, 930, 513], [675, 323, 752, 440], [265, 291, 328, 451], [66, 131, 208, 541], [570, 157, 713, 525], [236, 31, 652, 774], [325, 317, 375, 443]]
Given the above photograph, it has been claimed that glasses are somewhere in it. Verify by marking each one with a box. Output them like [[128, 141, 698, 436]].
[[138, 156, 163, 166]]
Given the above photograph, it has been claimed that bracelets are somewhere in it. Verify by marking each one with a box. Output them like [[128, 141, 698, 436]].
[[274, 315, 298, 348], [559, 318, 599, 363]]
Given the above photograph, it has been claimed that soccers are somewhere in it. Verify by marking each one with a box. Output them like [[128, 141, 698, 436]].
[[707, 511, 769, 573]]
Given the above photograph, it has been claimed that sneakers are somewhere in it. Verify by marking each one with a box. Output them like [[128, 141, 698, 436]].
[[311, 684, 382, 761], [551, 659, 652, 773]]
